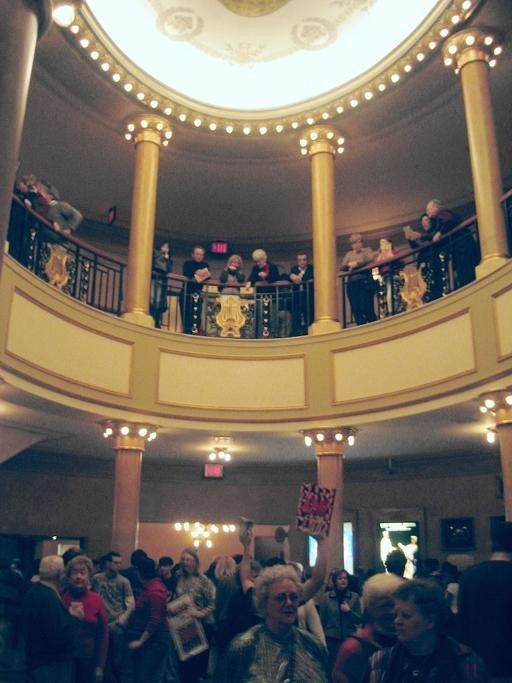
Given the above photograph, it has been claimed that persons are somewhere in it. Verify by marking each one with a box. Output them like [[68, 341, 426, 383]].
[[1, 518, 510, 683], [245, 248, 282, 338], [372, 236, 405, 316], [406, 197, 477, 292], [217, 253, 245, 295], [178, 245, 212, 335], [288, 251, 314, 336], [150, 240, 175, 329], [403, 212, 443, 305], [339, 231, 377, 326], [9, 170, 83, 288]]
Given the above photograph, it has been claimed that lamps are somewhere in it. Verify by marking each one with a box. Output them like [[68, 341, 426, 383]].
[[174, 521, 236, 551], [477, 390, 512, 412], [209, 447, 231, 461], [100, 422, 158, 442], [302, 426, 356, 447], [485, 427, 496, 442]]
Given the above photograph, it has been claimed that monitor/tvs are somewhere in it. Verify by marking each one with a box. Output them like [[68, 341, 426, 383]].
[[440, 517, 476, 551], [490, 515, 512, 552]]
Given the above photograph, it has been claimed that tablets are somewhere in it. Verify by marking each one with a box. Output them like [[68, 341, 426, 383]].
[[403, 226, 417, 240]]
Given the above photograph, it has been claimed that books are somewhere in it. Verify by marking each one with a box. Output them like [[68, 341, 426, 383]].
[[193, 267, 211, 283], [292, 478, 337, 539], [403, 225, 416, 241], [378, 241, 393, 260]]
